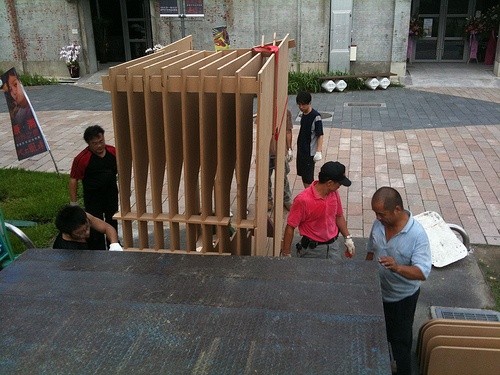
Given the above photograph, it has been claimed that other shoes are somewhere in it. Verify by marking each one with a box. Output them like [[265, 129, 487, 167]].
[[283, 201, 292, 211], [268, 200, 274, 211]]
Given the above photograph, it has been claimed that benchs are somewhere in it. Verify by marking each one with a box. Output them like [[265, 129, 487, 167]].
[[318, 72, 397, 93]]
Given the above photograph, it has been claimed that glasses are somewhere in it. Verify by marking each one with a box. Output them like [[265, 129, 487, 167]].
[[69, 217, 92, 240]]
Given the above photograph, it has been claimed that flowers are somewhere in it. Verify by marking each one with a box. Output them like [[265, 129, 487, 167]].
[[465, 4, 500, 34], [60, 43, 81, 69], [145, 44, 163, 55], [408, 12, 430, 38]]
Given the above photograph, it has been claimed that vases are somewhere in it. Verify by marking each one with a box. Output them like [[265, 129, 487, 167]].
[[68, 69, 80, 78]]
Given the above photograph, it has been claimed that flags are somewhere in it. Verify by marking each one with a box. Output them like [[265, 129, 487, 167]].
[[0, 67, 49, 160], [212, 27, 230, 51]]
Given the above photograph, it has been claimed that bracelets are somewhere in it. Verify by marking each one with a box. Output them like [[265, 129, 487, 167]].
[[344, 234, 353, 239]]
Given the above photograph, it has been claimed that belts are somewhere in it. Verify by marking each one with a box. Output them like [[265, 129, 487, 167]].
[[317, 231, 340, 245]]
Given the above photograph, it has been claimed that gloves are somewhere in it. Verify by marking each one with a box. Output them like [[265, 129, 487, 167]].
[[287, 148, 293, 162], [109, 243, 124, 251], [313, 152, 322, 163], [344, 238, 356, 257]]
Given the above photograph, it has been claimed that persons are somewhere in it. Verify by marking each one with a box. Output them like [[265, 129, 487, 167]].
[[71, 125, 122, 248], [268, 110, 293, 210], [7, 70, 33, 123], [53, 206, 123, 251], [367, 187, 432, 375], [296, 91, 324, 188], [281, 161, 355, 258]]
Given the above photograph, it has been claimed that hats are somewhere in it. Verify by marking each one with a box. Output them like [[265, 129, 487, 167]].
[[319, 161, 351, 187]]
